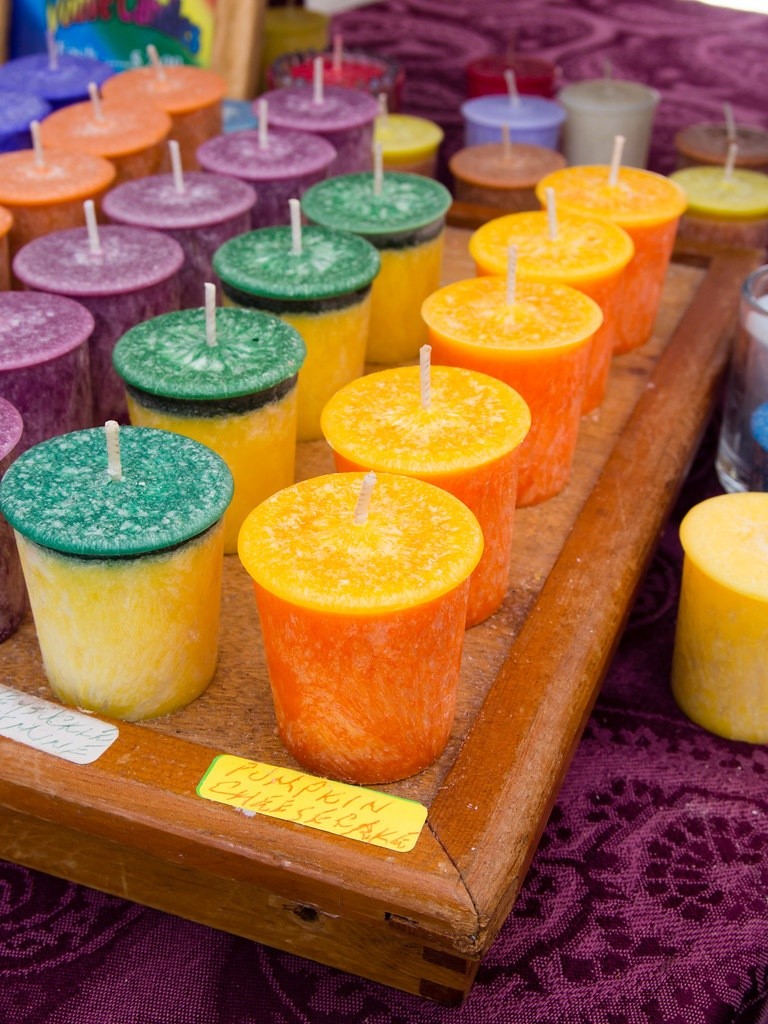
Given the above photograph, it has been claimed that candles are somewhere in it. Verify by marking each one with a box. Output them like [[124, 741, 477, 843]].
[[0, 7, 768, 789]]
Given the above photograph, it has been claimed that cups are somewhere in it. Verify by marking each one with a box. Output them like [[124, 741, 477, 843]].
[[0, 21, 768, 783]]
